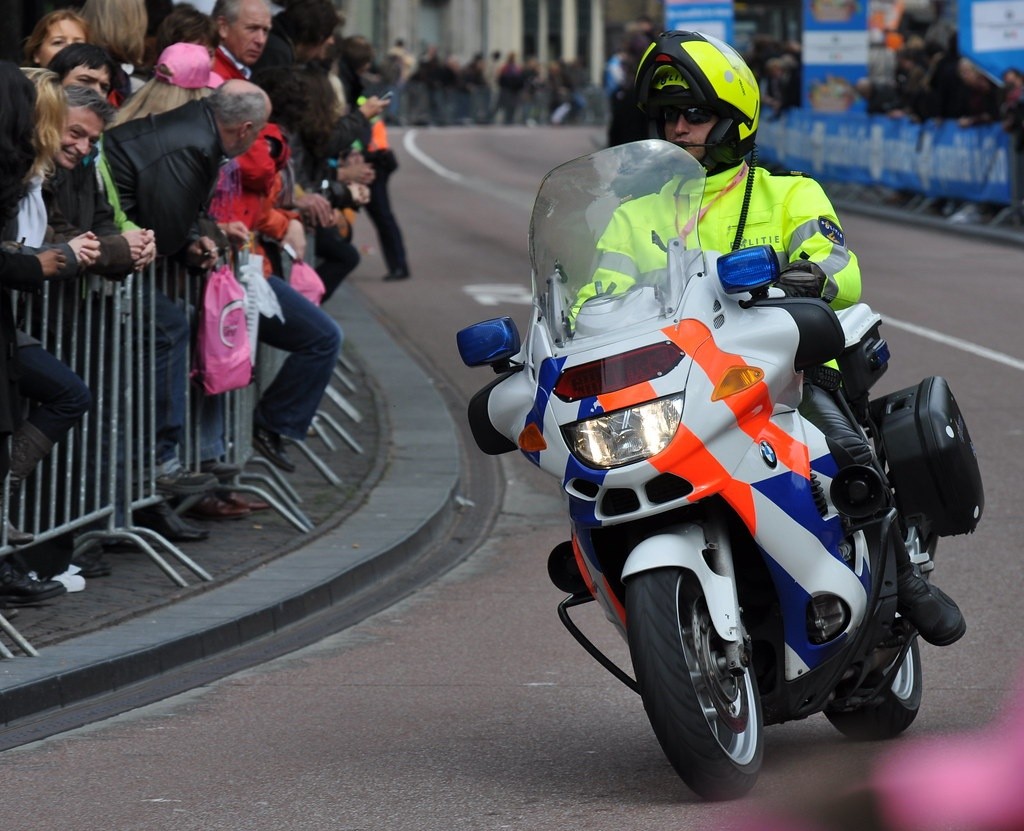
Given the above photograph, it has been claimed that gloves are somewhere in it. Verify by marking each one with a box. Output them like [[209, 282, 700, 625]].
[[773, 260, 826, 297]]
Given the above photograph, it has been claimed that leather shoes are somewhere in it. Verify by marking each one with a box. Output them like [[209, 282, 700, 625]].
[[227, 492, 272, 512], [184, 496, 253, 522], [147, 502, 210, 542], [0, 560, 67, 609], [252, 427, 296, 474]]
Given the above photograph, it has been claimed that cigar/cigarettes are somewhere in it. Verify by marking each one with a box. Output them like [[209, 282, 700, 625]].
[[205, 247, 218, 255]]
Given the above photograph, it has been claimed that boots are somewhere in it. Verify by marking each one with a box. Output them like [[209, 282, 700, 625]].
[[0, 419, 53, 545]]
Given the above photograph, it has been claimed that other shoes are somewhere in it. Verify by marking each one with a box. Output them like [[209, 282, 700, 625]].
[[72, 555, 111, 578], [901, 572, 967, 647], [383, 269, 409, 280], [195, 461, 240, 480]]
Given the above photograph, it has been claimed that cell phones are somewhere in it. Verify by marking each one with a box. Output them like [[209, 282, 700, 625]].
[[380, 91, 393, 99]]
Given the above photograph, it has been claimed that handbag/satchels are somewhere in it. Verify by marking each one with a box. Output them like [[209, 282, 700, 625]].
[[289, 262, 326, 308], [189, 265, 257, 397]]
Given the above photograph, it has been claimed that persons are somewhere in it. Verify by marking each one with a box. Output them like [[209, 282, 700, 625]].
[[565, 29, 966, 645], [0, 0, 1024, 597]]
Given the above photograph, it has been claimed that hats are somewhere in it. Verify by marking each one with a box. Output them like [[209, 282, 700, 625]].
[[155, 42, 224, 90]]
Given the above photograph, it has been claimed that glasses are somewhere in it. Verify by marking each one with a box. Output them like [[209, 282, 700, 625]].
[[663, 104, 712, 125]]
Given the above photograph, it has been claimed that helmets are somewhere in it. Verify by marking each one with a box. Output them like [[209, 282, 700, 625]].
[[633, 29, 760, 166]]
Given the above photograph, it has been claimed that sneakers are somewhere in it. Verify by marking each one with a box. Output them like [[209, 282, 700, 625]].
[[156, 466, 219, 493]]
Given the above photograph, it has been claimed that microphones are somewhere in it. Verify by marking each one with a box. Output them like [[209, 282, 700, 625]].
[[675, 141, 716, 151]]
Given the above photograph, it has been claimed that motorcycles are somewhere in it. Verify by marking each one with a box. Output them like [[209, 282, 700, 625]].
[[455, 136, 986, 805]]
